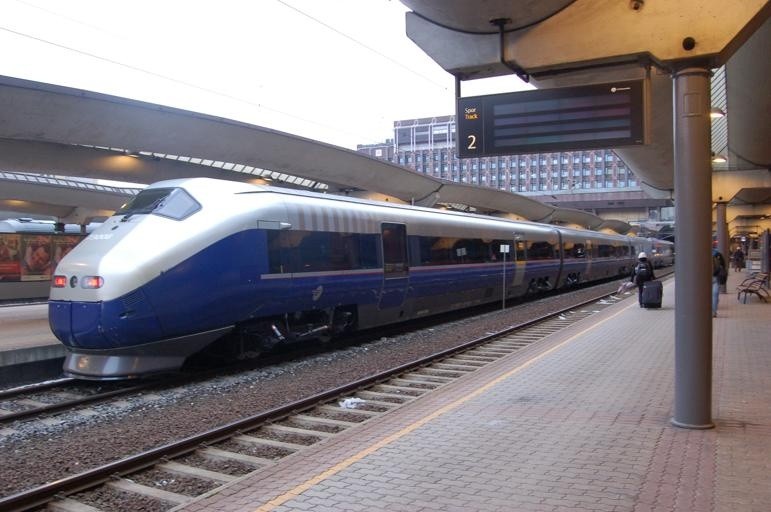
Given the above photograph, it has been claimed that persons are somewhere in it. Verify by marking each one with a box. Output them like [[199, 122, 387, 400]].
[[712, 239, 725, 318], [630, 251, 656, 309], [734, 247, 744, 272], [1, 234, 78, 273]]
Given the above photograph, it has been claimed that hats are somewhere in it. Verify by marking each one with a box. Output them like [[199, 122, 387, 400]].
[[637, 251, 648, 260]]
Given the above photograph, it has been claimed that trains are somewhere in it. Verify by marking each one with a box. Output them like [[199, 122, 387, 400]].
[[45, 173, 674, 391]]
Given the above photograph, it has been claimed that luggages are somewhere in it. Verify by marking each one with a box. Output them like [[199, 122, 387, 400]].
[[638, 278, 665, 309]]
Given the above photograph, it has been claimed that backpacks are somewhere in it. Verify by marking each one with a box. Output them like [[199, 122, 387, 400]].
[[636, 261, 651, 286], [712, 252, 721, 277]]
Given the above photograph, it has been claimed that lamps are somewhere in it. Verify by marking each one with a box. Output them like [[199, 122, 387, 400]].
[[710, 106, 725, 121], [712, 154, 727, 165]]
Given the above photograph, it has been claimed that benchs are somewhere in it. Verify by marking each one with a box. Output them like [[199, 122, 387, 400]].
[[737, 273, 770, 305]]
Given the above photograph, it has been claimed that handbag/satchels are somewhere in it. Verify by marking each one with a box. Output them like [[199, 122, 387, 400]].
[[717, 264, 728, 285]]
